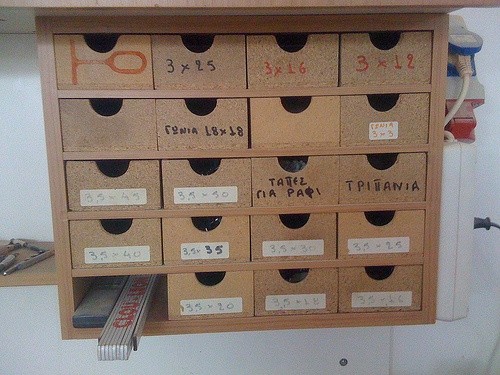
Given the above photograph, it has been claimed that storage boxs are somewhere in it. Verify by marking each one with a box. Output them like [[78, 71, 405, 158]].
[[30, 10, 448, 332]]
